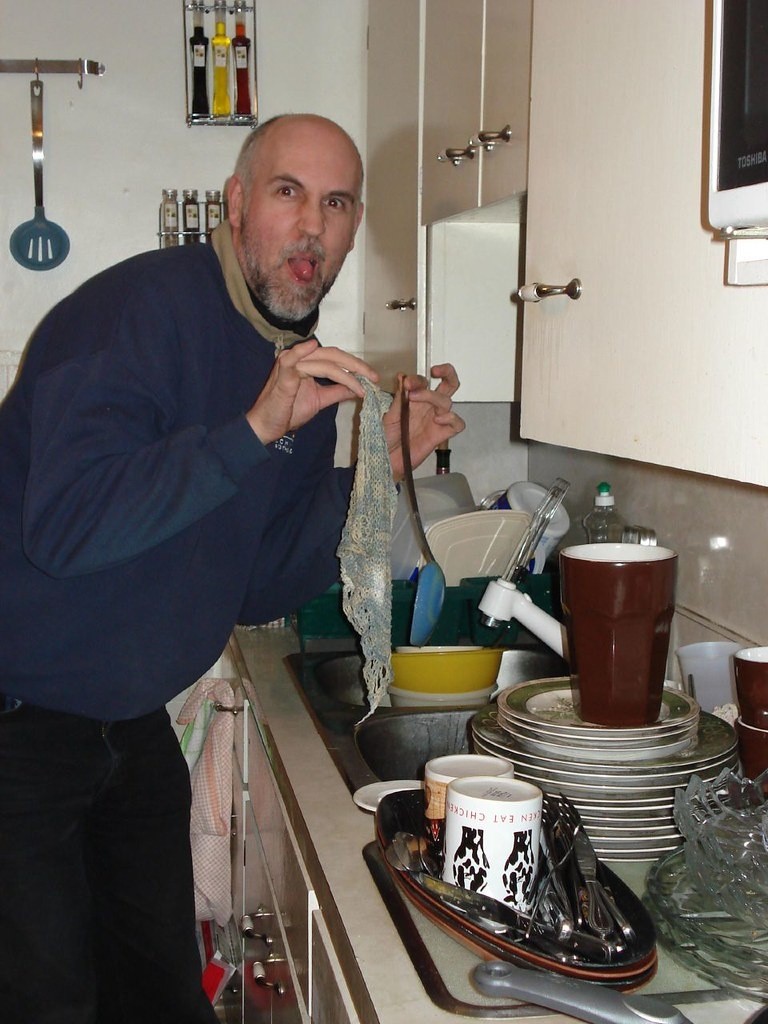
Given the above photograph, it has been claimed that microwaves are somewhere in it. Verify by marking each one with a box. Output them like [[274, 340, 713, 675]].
[[708, 0, 768, 235]]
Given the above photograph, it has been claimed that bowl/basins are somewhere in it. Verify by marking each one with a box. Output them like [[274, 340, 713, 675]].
[[384, 649, 503, 695], [395, 647, 482, 654]]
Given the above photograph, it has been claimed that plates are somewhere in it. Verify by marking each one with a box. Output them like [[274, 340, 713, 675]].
[[375, 788, 656, 969], [377, 836, 659, 993], [353, 779, 424, 812], [375, 821, 656, 978], [468, 674, 738, 863]]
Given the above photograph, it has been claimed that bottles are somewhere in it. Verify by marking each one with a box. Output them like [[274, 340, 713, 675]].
[[181, 189, 200, 246], [160, 188, 179, 249], [189, 0, 208, 122], [230, 0, 252, 120], [582, 482, 626, 544], [210, 0, 232, 121], [205, 190, 221, 245]]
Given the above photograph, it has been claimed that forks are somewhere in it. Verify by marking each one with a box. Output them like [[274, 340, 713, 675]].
[[537, 786, 637, 955]]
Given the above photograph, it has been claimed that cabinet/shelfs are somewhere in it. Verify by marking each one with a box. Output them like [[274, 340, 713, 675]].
[[362, 1, 530, 403], [160, 680, 390, 1023]]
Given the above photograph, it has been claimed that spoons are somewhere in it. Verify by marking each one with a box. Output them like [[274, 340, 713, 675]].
[[386, 832, 443, 882]]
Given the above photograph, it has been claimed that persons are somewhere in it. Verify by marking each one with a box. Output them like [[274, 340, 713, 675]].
[[0, 115, 465, 1024]]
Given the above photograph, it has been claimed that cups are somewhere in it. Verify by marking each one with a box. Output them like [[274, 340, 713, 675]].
[[557, 542, 677, 728], [672, 641, 768, 806], [424, 758, 516, 844], [441, 777, 543, 933]]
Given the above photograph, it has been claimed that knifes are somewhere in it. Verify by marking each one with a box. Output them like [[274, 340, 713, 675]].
[[418, 871, 613, 964]]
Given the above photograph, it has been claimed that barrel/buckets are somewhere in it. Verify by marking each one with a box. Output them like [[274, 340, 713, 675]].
[[487, 481, 569, 562], [387, 682, 499, 710]]
